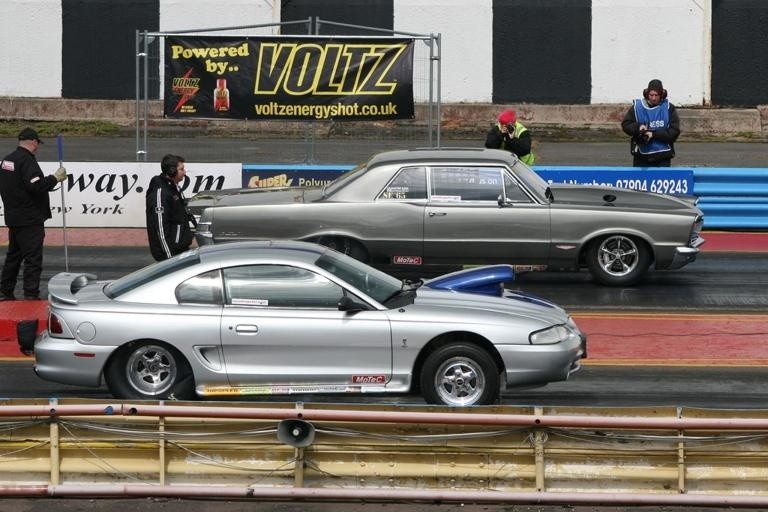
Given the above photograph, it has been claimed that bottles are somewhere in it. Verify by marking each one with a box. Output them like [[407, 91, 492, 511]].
[[212, 79, 230, 112]]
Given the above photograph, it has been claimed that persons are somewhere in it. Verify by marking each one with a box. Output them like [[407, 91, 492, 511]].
[[485, 111, 534, 167], [621, 80, 680, 166], [0, 128, 66, 301], [146, 155, 199, 261]]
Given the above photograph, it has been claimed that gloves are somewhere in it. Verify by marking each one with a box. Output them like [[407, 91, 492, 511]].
[[52, 167, 67, 181]]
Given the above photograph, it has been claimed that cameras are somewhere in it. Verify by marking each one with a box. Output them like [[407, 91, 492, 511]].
[[506, 125, 514, 133]]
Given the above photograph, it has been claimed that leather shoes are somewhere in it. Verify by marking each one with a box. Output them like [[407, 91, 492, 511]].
[[0, 292, 40, 301]]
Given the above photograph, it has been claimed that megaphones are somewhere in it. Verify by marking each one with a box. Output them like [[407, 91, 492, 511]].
[[277, 419, 315, 448]]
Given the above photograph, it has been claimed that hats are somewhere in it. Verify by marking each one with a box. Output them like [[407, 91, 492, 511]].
[[649, 80, 662, 92], [19, 127, 44, 144], [498, 110, 516, 125]]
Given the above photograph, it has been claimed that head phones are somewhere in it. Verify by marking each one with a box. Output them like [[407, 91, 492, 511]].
[[643, 79, 667, 99], [162, 160, 177, 177]]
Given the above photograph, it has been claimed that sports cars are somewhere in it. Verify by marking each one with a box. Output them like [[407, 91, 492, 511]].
[[25, 238, 587, 407], [183, 146, 707, 288]]
